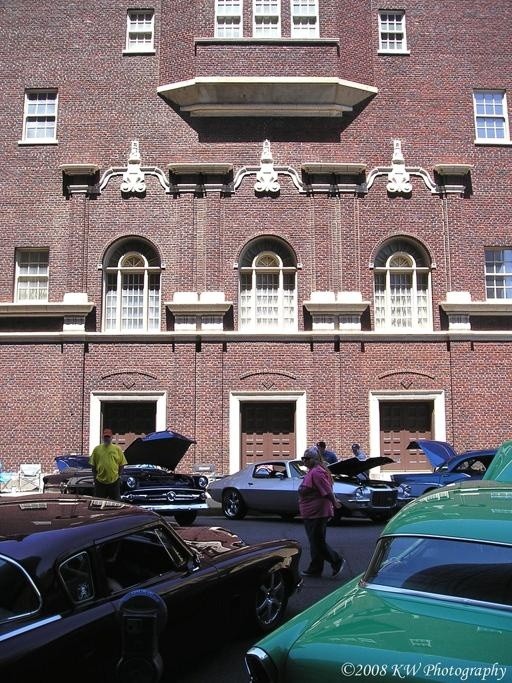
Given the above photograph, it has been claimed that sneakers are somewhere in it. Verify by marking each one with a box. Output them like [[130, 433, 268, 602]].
[[331, 559, 345, 578], [302, 571, 319, 576]]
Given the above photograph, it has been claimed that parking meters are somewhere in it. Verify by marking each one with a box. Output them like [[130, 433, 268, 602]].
[[109, 584, 169, 682]]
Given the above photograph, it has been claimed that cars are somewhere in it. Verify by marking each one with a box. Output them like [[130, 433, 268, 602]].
[[39, 427, 212, 528], [392, 436, 501, 505], [206, 452, 408, 528], [239, 437, 512, 682], [0, 489, 306, 681]]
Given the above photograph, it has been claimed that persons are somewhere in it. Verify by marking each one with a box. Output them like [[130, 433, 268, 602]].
[[88, 428, 128, 500], [297, 445, 347, 580], [352, 443, 368, 481], [316, 441, 338, 465]]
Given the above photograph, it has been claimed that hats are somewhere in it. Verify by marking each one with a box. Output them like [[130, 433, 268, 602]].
[[317, 442, 326, 447], [104, 429, 112, 437]]
